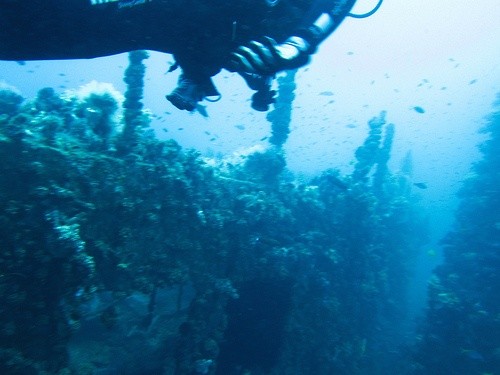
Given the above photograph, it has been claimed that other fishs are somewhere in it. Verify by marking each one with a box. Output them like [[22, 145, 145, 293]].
[[17, 46, 480, 192]]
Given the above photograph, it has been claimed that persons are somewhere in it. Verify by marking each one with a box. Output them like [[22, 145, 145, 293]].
[[0, 0, 357, 76]]
